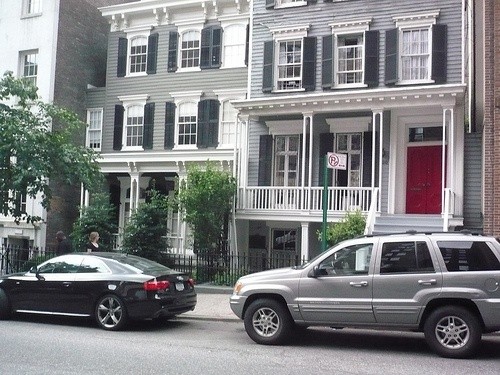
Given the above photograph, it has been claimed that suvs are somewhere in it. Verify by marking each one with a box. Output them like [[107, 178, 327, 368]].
[[229, 231, 500, 360]]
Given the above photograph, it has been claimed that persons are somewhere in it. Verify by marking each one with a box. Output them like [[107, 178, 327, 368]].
[[86, 232, 106, 253], [55, 230, 73, 256]]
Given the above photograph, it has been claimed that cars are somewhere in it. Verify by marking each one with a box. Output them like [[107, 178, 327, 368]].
[[1, 251, 197, 332]]
